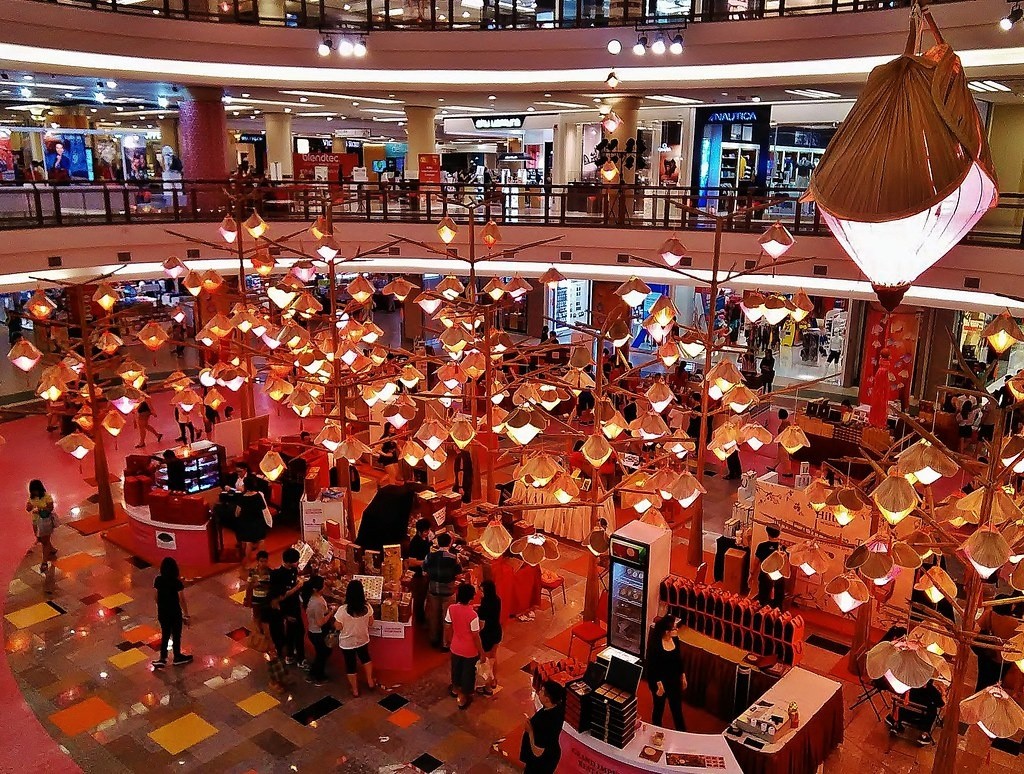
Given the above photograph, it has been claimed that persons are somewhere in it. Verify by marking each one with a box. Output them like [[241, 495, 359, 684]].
[[221, 422, 503, 710], [171, 323, 187, 358], [842, 399, 853, 411], [440, 166, 468, 198], [151, 557, 194, 668], [534, 169, 541, 184], [45, 381, 83, 434], [163, 450, 186, 490], [748, 523, 790, 611], [26, 480, 58, 571], [174, 404, 234, 445], [48, 144, 70, 170], [5, 314, 22, 343], [520, 681, 567, 774], [162, 146, 183, 196], [957, 369, 1024, 458], [883, 625, 945, 745], [647, 616, 688, 731], [484, 167, 492, 191], [541, 326, 794, 480], [380, 169, 389, 189], [134, 400, 163, 448]]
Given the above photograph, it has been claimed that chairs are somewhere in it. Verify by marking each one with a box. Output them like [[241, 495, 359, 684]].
[[847, 643, 891, 722], [534, 559, 566, 614], [568, 588, 609, 667]]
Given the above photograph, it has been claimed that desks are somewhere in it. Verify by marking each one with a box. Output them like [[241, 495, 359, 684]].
[[721, 665, 843, 757]]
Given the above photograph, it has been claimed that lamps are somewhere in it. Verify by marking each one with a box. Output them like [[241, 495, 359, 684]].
[[6, 6, 1024, 738]]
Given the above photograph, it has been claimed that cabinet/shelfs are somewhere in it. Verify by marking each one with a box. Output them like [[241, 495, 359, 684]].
[[717, 146, 760, 216], [566, 181, 645, 212]]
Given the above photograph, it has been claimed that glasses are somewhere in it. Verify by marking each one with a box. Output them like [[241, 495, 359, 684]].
[[670, 628, 679, 631]]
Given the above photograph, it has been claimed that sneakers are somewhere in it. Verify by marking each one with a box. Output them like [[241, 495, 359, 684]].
[[172, 654, 193, 665], [151, 660, 167, 668]]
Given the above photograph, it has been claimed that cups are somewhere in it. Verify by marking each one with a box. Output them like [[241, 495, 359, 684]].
[[298, 576, 303, 586], [253, 575, 259, 584]]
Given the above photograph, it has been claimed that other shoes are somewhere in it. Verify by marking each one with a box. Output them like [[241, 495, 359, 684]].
[[176, 352, 183, 357], [448, 684, 458, 698], [723, 474, 738, 479], [50, 548, 57, 556], [782, 473, 793, 477], [351, 688, 359, 698], [40, 562, 48, 570], [367, 679, 375, 690], [765, 466, 775, 472], [476, 686, 494, 696], [457, 696, 472, 710], [133, 429, 202, 448]]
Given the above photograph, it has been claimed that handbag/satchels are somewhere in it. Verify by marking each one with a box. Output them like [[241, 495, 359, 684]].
[[258, 491, 273, 528], [36, 516, 53, 537], [325, 629, 340, 647], [475, 657, 496, 681], [51, 509, 60, 528]]
